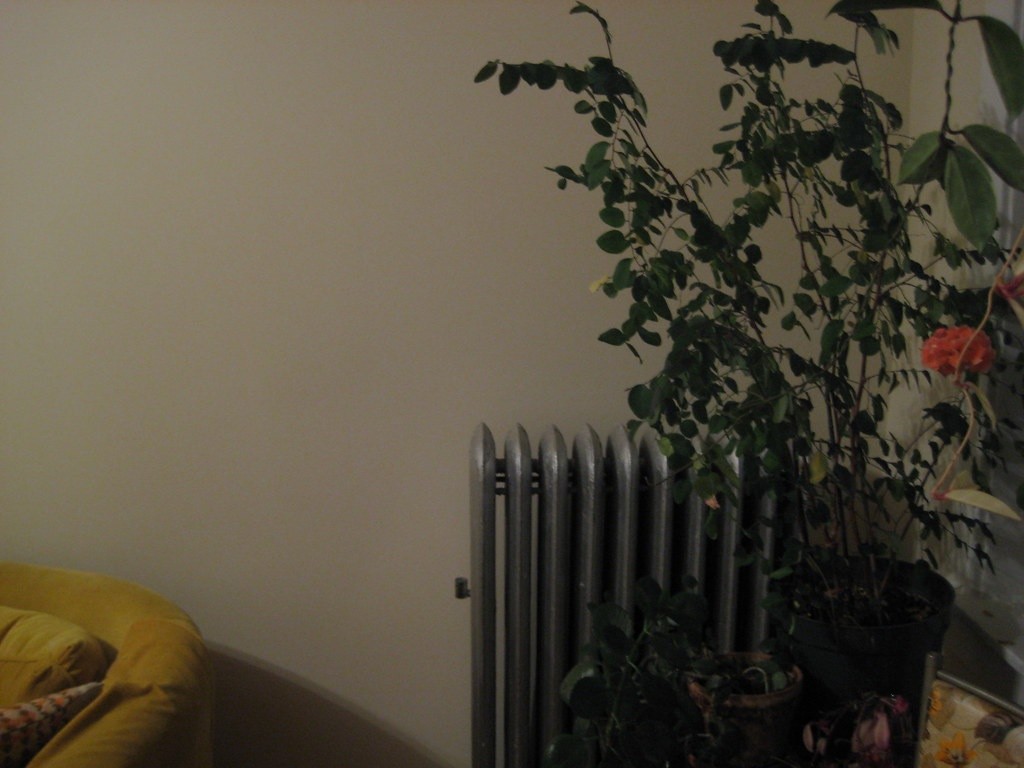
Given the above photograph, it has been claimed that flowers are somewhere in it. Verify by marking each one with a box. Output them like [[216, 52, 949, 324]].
[[913, 318, 1002, 386]]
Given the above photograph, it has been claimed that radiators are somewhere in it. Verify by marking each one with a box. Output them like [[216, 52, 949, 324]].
[[450, 417, 810, 768]]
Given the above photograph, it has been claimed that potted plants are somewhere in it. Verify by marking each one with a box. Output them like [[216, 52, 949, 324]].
[[476, 0, 1024, 768]]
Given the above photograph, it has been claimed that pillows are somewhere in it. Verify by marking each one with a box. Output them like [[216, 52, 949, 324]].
[[0, 603, 108, 768]]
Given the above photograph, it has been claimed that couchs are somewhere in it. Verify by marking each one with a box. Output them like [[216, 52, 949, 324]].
[[0, 555, 217, 768]]
